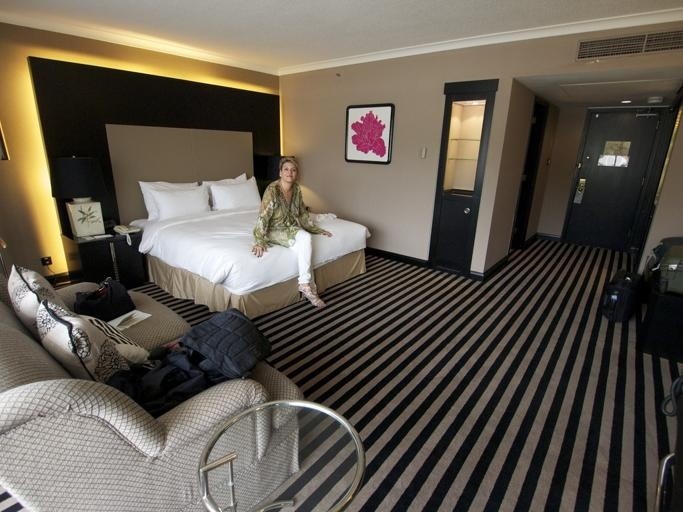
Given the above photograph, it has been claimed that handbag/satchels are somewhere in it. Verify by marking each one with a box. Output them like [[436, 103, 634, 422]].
[[74, 276, 136, 323]]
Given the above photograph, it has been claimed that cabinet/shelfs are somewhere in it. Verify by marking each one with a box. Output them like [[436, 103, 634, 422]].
[[427, 78, 500, 279], [636, 268, 682, 363]]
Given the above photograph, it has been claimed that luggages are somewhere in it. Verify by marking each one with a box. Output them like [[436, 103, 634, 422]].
[[601, 270, 644, 321], [651, 237, 683, 294]]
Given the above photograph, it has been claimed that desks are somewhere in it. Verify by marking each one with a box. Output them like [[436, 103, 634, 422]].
[[195, 399, 366, 512]]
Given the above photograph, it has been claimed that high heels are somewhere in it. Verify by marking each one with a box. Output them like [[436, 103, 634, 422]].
[[298, 284, 326, 307]]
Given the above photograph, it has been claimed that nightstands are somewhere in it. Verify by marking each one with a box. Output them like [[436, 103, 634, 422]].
[[73, 227, 148, 288]]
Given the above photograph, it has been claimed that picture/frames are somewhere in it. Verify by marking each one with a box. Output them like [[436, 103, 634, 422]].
[[344, 101, 394, 166]]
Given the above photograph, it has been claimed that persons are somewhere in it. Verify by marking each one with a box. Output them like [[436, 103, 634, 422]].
[[253, 156, 333, 309]]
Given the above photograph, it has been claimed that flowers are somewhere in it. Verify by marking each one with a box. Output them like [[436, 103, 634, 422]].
[[351, 110, 387, 158]]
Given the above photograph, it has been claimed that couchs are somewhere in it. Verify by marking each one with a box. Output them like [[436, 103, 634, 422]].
[[1, 265, 305, 512]]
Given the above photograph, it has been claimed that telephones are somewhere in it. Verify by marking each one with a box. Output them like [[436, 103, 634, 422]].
[[113, 224, 141, 235]]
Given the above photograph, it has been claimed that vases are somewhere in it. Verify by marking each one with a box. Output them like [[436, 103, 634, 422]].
[[65, 195, 107, 239]]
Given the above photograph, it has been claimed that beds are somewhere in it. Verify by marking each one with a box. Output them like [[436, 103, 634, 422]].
[[128, 174, 370, 317]]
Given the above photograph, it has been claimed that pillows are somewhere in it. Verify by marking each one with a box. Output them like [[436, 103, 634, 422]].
[[81, 314, 152, 366], [6, 264, 71, 336], [137, 173, 265, 222], [36, 299, 131, 384]]
